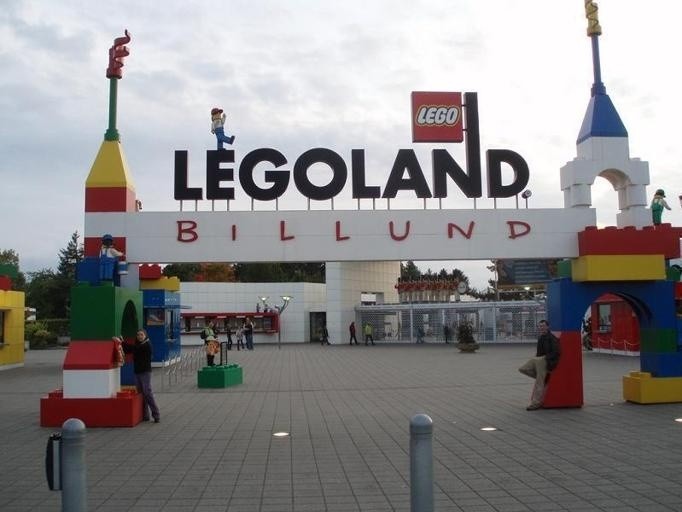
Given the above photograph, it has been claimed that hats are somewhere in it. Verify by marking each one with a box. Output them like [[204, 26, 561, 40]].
[[205, 335, 216, 341]]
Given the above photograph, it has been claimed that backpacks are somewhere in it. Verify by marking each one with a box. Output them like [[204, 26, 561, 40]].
[[200, 327, 210, 339]]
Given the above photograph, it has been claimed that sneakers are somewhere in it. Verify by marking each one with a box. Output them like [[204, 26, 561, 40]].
[[526, 401, 540, 412], [144, 417, 160, 423]]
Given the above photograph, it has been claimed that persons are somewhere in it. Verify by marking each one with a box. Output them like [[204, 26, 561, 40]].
[[112, 329, 161, 423], [416, 325, 424, 344], [444, 325, 449, 344], [202, 317, 254, 366], [98, 234, 124, 281], [518, 321, 561, 411], [320, 323, 331, 345], [650, 190, 671, 224], [211, 108, 235, 150], [364, 323, 376, 345], [583, 316, 592, 351], [349, 322, 359, 345]]
